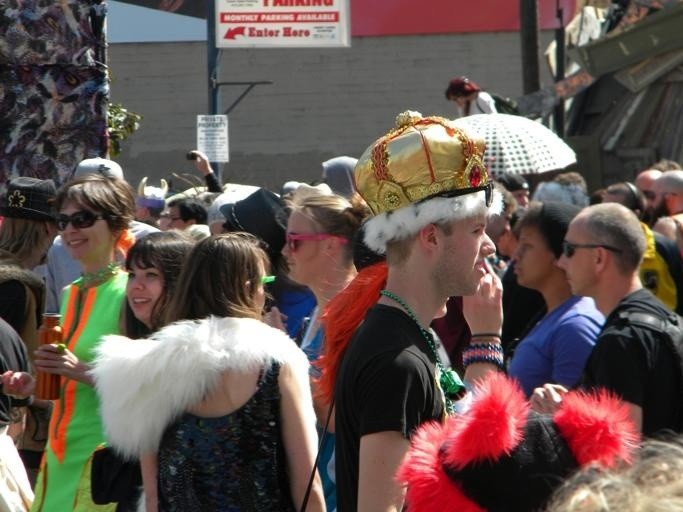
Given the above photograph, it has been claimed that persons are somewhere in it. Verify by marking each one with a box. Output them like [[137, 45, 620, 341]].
[[0, 76, 683, 512]]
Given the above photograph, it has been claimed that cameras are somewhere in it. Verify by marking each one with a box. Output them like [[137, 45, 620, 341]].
[[186, 152, 196, 161]]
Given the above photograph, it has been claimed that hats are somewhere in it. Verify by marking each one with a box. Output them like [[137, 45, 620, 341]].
[[0, 177, 57, 223], [71, 156, 123, 181], [496, 174, 528, 191], [354, 110, 502, 272], [136, 177, 168, 209], [395, 370, 641, 511], [219, 187, 292, 268]]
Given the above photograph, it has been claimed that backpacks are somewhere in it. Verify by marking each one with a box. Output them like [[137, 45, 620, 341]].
[[602, 310, 682, 433]]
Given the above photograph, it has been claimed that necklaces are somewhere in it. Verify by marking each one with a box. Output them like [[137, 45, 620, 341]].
[[380, 290, 464, 415]]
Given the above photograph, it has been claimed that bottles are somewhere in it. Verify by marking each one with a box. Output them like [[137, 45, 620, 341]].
[[35, 312, 62, 400]]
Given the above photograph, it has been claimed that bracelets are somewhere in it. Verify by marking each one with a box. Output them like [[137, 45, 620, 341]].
[[470, 333, 502, 344], [462, 342, 504, 369]]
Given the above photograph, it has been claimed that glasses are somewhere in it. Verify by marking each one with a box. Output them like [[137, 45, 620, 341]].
[[285, 233, 350, 254], [55, 209, 106, 230], [414, 179, 494, 207], [561, 240, 623, 259]]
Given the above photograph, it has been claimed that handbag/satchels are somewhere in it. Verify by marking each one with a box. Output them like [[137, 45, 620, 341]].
[[491, 93, 520, 116], [6, 406, 50, 451]]
